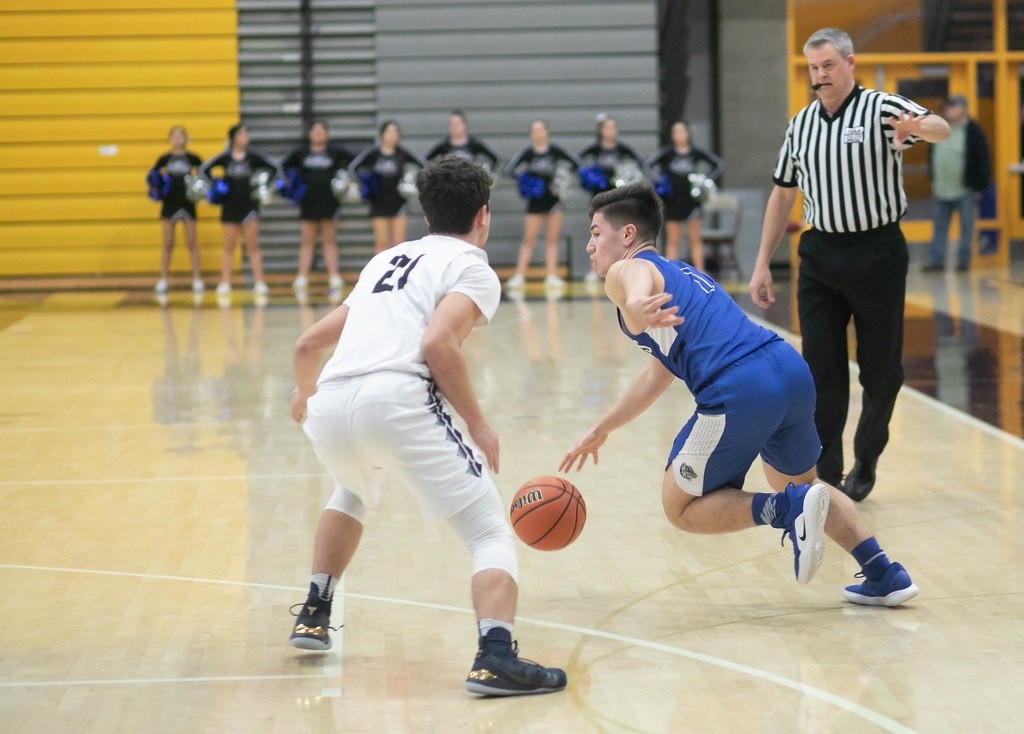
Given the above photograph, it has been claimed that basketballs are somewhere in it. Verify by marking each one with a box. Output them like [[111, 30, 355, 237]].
[[510, 474, 587, 552]]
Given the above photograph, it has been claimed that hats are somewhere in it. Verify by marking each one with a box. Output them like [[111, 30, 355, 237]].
[[943, 95, 967, 107]]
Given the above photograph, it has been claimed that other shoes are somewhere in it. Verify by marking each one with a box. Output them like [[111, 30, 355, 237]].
[[504, 275, 526, 299], [156, 280, 169, 294], [193, 279, 206, 291], [328, 277, 345, 296], [253, 281, 270, 296], [585, 272, 599, 290], [216, 282, 232, 295], [546, 276, 565, 291], [294, 277, 308, 296], [920, 260, 945, 273], [956, 263, 970, 272]]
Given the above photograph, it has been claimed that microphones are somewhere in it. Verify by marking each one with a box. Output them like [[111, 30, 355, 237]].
[[812, 84, 821, 90]]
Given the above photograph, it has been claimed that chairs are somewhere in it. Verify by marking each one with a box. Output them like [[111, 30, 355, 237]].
[[695, 195, 744, 280]]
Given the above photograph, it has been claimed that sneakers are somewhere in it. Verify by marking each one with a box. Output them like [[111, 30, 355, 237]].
[[290, 593, 333, 650], [464, 637, 568, 697], [770, 482, 830, 585], [842, 562, 919, 607]]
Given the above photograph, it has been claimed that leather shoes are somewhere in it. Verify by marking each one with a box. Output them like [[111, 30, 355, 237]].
[[837, 484, 845, 492], [845, 467, 875, 501]]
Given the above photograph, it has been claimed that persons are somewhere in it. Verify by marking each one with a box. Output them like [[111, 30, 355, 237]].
[[751, 22, 949, 506], [199, 122, 281, 295], [558, 177, 917, 610], [422, 112, 499, 187], [643, 117, 725, 273], [571, 113, 646, 296], [919, 92, 994, 273], [347, 119, 426, 259], [276, 121, 359, 291], [503, 118, 583, 304], [147, 123, 211, 295], [285, 156, 566, 696]]
